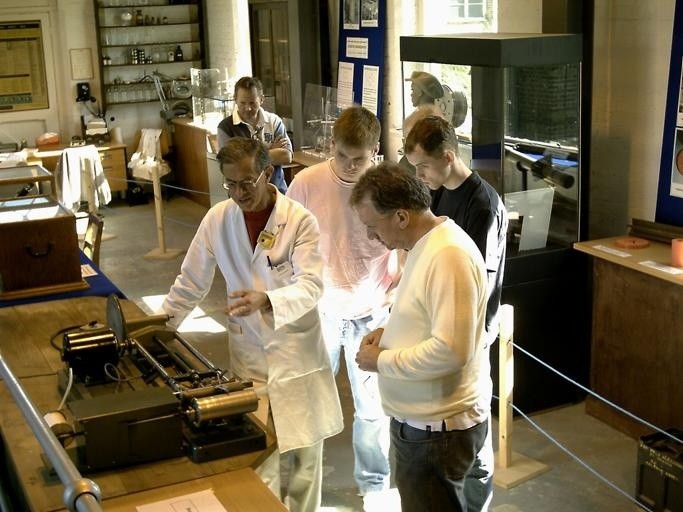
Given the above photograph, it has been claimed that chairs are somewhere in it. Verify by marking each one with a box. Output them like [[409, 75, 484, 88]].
[[81, 211, 104, 270]]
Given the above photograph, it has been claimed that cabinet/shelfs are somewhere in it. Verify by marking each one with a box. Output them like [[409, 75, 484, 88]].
[[170, 117, 230, 209], [0, 20, 49, 113], [1, 141, 128, 209], [93, 1, 211, 117]]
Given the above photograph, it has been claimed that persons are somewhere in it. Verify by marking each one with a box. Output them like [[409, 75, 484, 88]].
[[347, 160, 496, 512], [216, 76, 294, 195], [402, 114, 510, 511], [398, 71, 445, 139], [284, 105, 409, 512], [153, 135, 345, 512]]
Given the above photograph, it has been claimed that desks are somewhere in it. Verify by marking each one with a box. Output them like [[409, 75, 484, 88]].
[[0, 248, 289, 512], [574, 235, 683, 441]]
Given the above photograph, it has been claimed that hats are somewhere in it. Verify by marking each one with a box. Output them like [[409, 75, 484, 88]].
[[405, 71, 443, 99]]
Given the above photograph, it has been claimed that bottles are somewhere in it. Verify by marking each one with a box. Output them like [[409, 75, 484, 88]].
[[144, 14, 168, 25], [129, 44, 183, 65], [21, 138, 27, 149], [135, 9, 143, 26], [103, 54, 111, 66], [105, 84, 158, 103]]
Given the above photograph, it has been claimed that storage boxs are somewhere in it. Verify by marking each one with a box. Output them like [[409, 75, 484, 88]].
[[634, 428, 683, 512]]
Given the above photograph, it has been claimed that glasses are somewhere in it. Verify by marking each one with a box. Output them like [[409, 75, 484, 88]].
[[222, 170, 264, 190]]
